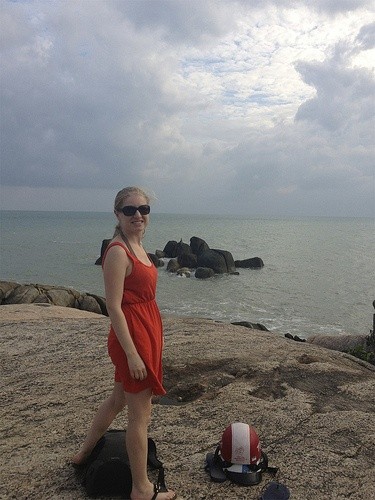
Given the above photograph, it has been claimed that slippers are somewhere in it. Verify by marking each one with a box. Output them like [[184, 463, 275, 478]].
[[257, 482, 290, 500], [127, 483, 177, 500], [72, 464, 85, 470], [204, 453, 226, 483]]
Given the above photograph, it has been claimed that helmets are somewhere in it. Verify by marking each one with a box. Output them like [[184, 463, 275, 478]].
[[218, 423, 261, 464]]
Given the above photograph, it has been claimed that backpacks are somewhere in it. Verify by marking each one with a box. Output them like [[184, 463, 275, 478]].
[[80, 430, 165, 495]]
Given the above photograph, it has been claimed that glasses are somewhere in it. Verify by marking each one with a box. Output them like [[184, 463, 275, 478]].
[[115, 205, 150, 216]]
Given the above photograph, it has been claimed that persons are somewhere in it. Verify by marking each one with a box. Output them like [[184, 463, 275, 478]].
[[73, 184, 176, 500]]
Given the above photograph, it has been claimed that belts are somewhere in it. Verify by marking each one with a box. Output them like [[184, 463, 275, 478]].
[[214, 444, 281, 486]]
